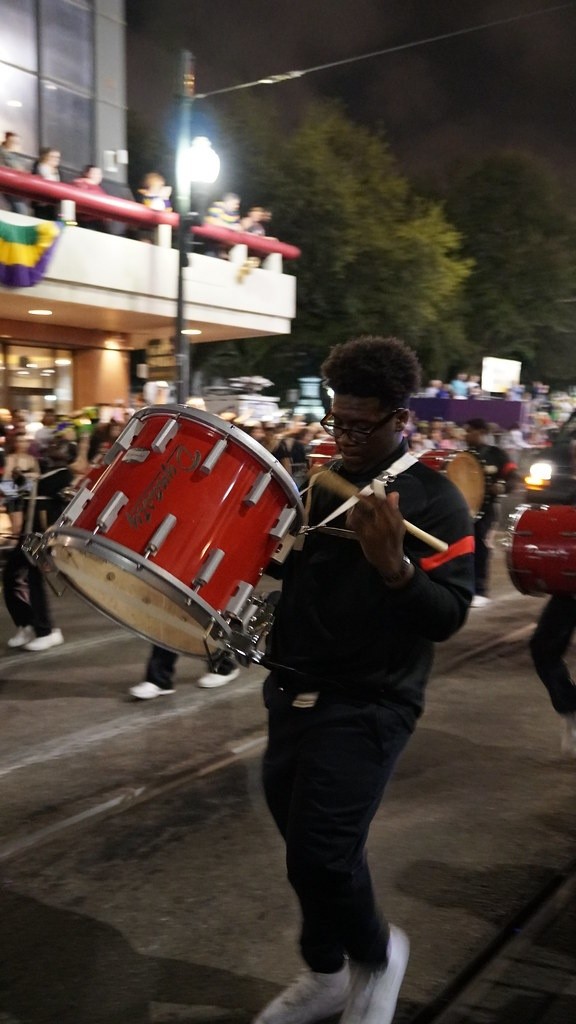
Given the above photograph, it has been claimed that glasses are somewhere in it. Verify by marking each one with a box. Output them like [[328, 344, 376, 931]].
[[321, 411, 399, 444]]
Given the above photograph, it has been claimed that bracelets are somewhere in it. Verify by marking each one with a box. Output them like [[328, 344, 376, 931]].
[[381, 554, 410, 584]]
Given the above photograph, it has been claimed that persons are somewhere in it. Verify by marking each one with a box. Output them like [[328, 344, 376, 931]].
[[529, 405, 576, 756], [129, 396, 334, 698], [0, 401, 141, 650], [256, 332, 476, 1024], [127, 173, 176, 241], [68, 165, 114, 236], [204, 191, 273, 269], [408, 370, 576, 609], [0, 131, 31, 214], [29, 145, 66, 221]]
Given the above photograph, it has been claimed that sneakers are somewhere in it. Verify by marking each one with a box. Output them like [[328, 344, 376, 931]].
[[256, 954, 349, 1024], [339, 923, 410, 1024]]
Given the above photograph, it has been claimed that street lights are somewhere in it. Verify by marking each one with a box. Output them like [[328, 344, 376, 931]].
[[174, 135, 222, 405]]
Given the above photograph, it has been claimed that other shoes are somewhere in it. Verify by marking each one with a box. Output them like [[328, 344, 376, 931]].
[[198, 668, 240, 688], [24, 628, 62, 651], [472, 596, 489, 607], [130, 681, 176, 699], [8, 625, 37, 647]]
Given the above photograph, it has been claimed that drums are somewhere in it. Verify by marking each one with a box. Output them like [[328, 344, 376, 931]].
[[502, 504, 576, 599], [309, 436, 343, 469], [415, 448, 486, 523], [40, 403, 308, 662]]
